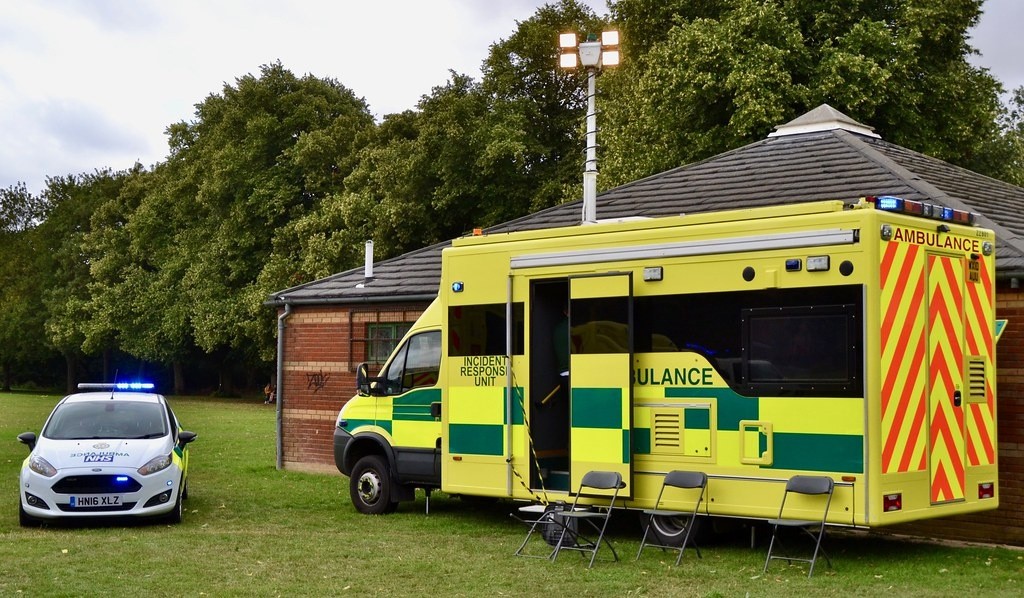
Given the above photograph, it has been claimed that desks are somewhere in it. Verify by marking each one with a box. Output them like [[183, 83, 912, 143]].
[[508, 500, 600, 559]]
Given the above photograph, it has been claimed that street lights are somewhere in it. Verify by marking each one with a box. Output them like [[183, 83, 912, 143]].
[[560, 29, 621, 225]]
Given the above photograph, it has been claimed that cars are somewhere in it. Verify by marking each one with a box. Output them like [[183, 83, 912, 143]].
[[16, 382, 198, 527]]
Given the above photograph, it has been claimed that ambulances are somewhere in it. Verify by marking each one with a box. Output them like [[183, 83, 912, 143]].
[[334, 195, 1000, 548]]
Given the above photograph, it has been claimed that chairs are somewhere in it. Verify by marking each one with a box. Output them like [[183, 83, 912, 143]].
[[635, 471, 708, 567], [763, 475, 834, 580], [551, 471, 622, 567]]
